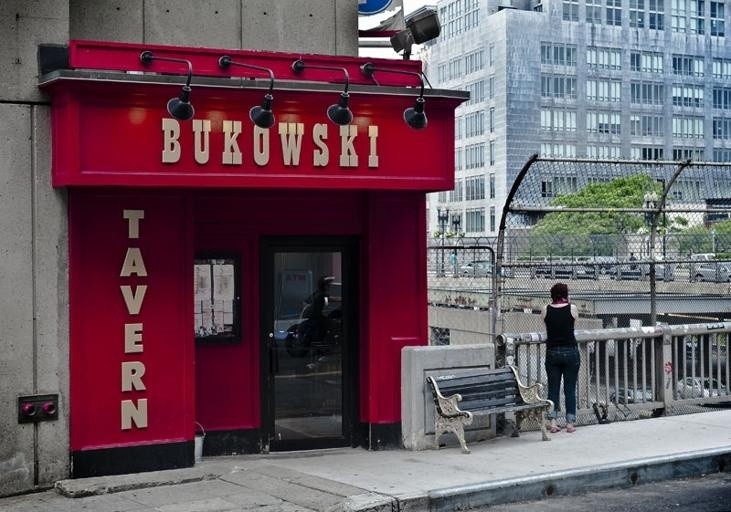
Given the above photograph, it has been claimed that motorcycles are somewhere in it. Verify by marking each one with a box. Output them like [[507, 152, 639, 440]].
[[286, 274, 341, 356]]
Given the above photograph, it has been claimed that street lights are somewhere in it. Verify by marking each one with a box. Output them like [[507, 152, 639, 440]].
[[436, 201, 449, 276], [641, 191, 667, 256], [450, 207, 463, 255]]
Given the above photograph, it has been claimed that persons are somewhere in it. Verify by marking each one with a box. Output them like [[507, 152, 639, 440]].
[[540, 283, 582, 433], [290, 309, 341, 379], [300, 273, 341, 321], [450, 252, 458, 274], [629, 252, 636, 271]]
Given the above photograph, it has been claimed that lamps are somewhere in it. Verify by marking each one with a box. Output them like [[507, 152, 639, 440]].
[[139, 49, 429, 130]]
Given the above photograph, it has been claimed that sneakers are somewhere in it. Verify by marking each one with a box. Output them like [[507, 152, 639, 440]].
[[565, 425, 575, 433], [550, 424, 561, 433]]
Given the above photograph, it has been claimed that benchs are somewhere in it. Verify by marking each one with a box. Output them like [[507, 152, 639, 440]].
[[425, 365, 554, 453]]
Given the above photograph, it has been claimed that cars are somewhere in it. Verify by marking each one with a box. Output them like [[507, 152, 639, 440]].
[[678, 376, 729, 397], [690, 254, 731, 283], [530, 255, 674, 279], [610, 388, 652, 403], [463, 260, 514, 277]]
